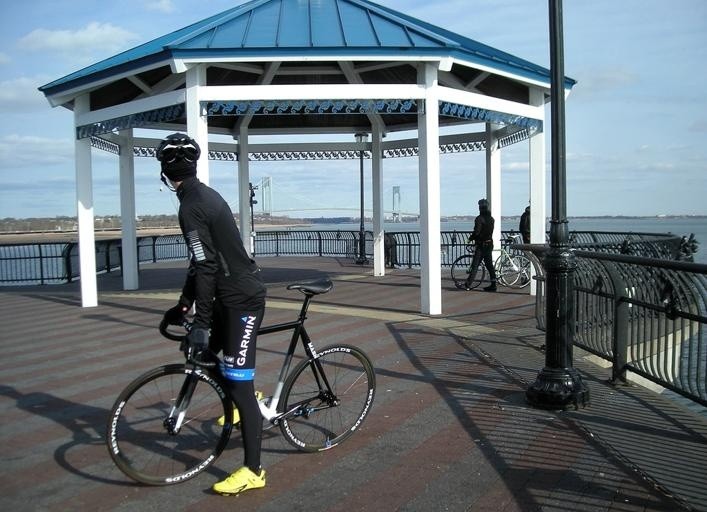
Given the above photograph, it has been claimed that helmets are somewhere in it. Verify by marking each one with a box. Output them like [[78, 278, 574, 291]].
[[156, 133, 200, 163], [478, 199, 490, 205]]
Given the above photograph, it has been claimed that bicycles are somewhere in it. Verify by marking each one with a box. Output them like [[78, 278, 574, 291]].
[[494, 235, 531, 287], [451, 238, 531, 290], [106, 277, 377, 486]]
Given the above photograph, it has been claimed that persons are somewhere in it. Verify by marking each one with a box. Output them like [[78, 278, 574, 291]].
[[157, 133, 268, 497], [520, 200, 531, 244], [456, 198, 498, 292]]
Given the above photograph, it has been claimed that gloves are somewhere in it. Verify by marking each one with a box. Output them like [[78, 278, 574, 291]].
[[185, 328, 210, 350], [164, 304, 190, 326]]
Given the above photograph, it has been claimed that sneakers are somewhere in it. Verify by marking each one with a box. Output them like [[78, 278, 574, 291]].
[[213, 466, 266, 497], [218, 391, 262, 425], [483, 286, 497, 291]]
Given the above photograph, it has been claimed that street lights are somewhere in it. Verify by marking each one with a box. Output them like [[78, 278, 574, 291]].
[[354, 133, 369, 265]]
